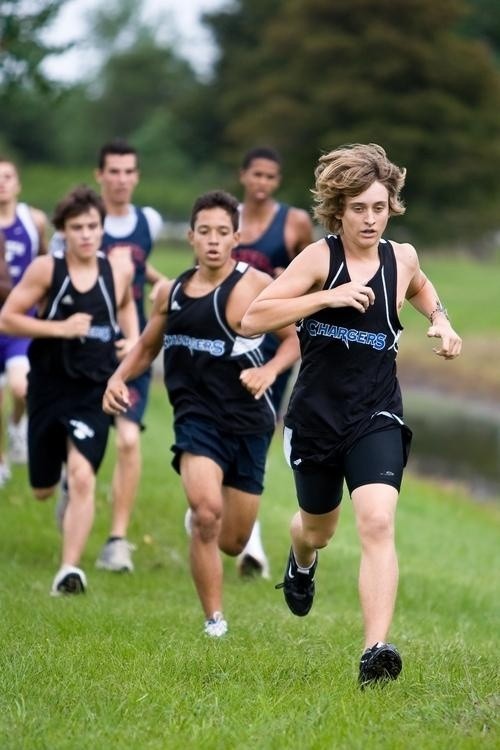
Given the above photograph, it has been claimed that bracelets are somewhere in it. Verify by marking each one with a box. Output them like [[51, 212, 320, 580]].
[[430, 308, 449, 324]]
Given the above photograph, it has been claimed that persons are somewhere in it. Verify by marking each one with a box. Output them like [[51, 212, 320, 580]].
[[103, 190, 300, 637], [0, 184, 140, 596], [241, 142, 462, 694], [194, 145, 313, 582], [0, 159, 49, 485], [50, 147, 171, 576]]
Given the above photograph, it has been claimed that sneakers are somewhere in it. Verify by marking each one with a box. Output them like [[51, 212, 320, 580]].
[[51, 564, 88, 594], [274, 545, 319, 617], [0, 462, 11, 484], [237, 544, 268, 583], [357, 641, 402, 691], [94, 540, 138, 573], [203, 611, 230, 639], [8, 416, 27, 463]]
